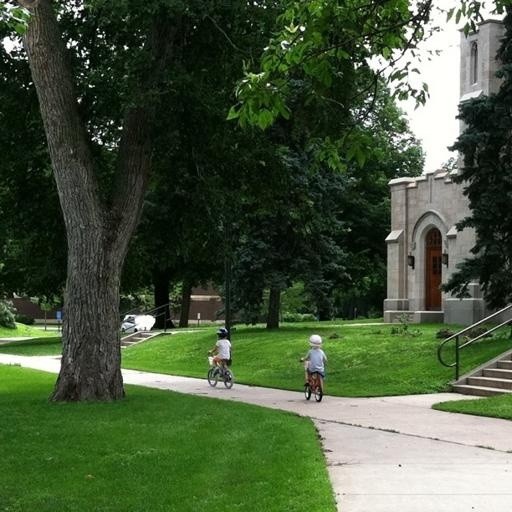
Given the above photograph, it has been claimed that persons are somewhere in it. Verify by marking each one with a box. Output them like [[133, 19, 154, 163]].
[[206, 326, 232, 379], [300, 334, 328, 396]]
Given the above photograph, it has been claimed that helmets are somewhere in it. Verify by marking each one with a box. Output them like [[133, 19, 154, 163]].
[[216, 327, 228, 334]]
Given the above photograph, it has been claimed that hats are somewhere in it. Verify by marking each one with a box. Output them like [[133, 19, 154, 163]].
[[308, 334, 323, 347]]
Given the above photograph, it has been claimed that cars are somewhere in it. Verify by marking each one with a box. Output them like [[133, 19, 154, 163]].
[[120, 314, 155, 334]]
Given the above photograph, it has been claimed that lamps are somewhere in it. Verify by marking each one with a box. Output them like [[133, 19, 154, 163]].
[[407, 252, 414, 269], [442, 249, 448, 268]]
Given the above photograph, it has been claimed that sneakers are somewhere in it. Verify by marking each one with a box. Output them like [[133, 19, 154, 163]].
[[304, 383, 310, 387], [212, 367, 219, 375]]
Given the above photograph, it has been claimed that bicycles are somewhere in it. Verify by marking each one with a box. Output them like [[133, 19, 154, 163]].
[[299, 357, 323, 402], [207, 350, 234, 390]]
